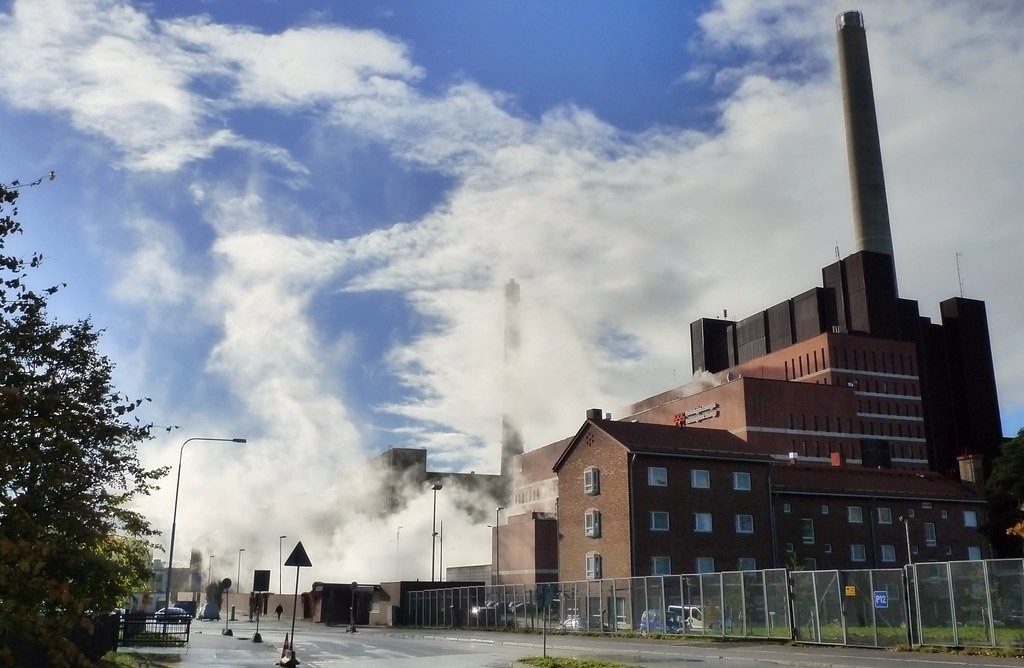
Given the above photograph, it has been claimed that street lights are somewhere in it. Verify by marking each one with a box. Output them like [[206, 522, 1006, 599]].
[[431, 485, 443, 581], [237, 549, 245, 593], [496, 507, 504, 585], [162, 438, 247, 635], [280, 536, 287, 594], [208, 556, 215, 586], [899, 515, 910, 565]]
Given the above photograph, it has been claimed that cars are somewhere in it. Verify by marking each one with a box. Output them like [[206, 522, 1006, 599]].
[[556, 617, 608, 629], [472, 601, 535, 615], [154, 608, 193, 624]]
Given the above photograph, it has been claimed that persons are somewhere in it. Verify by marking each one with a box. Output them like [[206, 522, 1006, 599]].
[[275, 603, 284, 621]]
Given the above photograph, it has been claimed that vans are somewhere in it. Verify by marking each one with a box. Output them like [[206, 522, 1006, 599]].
[[668, 604, 721, 632], [639, 609, 683, 636]]
